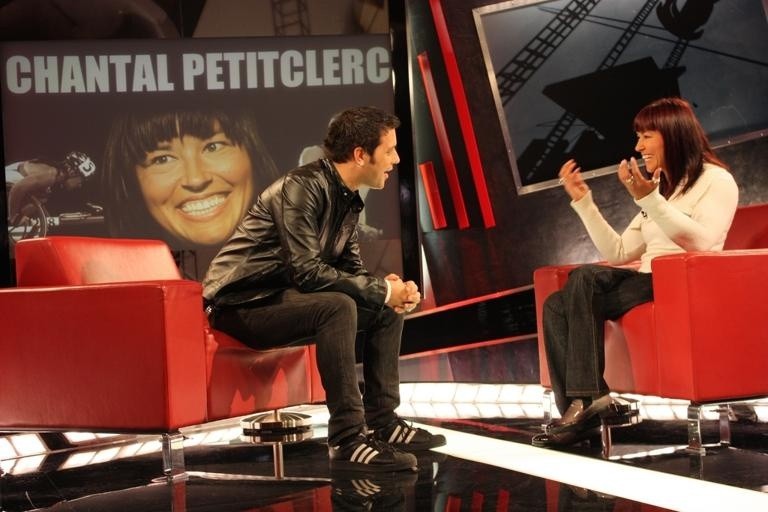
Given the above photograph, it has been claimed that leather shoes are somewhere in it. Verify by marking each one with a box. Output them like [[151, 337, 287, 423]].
[[531, 395, 618, 447]]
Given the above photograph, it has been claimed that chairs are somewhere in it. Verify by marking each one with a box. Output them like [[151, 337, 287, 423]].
[[1, 235, 328, 493], [532, 200, 768, 462]]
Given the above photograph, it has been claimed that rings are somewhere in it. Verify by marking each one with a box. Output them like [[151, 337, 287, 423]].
[[559, 178, 565, 185], [652, 177, 660, 183], [626, 176, 634, 185]]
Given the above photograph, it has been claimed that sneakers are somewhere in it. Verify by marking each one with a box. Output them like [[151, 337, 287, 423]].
[[330, 473, 419, 512], [328, 418, 446, 473]]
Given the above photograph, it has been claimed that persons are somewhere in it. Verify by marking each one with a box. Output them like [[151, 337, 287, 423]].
[[5, 151, 96, 226], [101, 99, 280, 249], [530, 97, 740, 447], [201, 105, 446, 472]]
